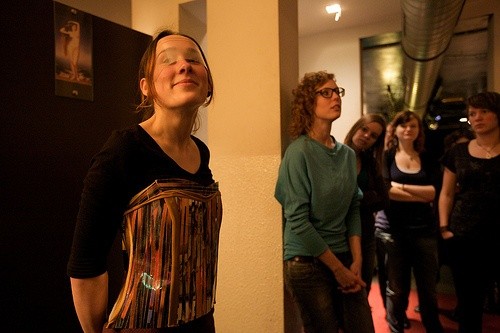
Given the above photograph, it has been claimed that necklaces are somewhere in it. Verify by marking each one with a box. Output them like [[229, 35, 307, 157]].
[[398, 153, 414, 169], [477, 142, 498, 159]]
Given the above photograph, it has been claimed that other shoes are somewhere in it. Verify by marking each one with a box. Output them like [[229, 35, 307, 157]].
[[403, 314, 410, 329]]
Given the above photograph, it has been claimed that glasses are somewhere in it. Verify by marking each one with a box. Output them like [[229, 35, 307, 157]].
[[315, 87, 345, 99]]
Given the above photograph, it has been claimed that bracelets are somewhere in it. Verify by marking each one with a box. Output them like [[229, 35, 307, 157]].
[[400, 183, 403, 191], [441, 226, 448, 234]]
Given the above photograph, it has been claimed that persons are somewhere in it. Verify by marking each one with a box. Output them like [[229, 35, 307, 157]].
[[372, 111, 445, 333], [275, 70, 375, 333], [440, 92, 500, 333], [337, 116, 386, 300], [68, 30, 223, 333]]
